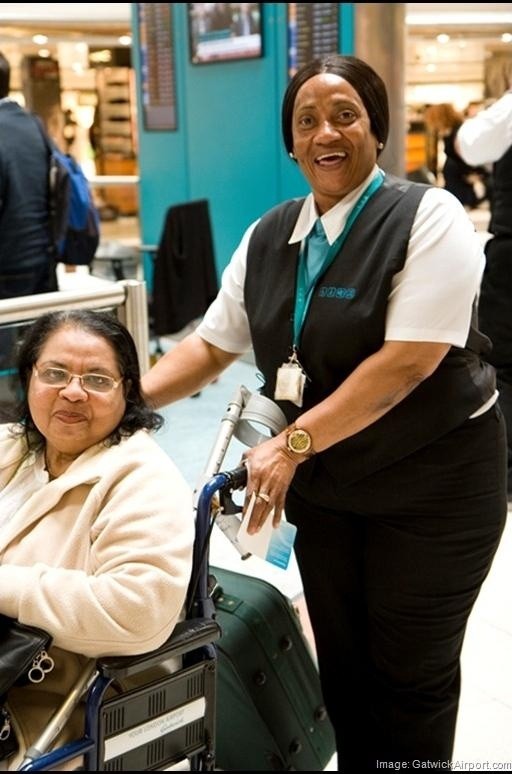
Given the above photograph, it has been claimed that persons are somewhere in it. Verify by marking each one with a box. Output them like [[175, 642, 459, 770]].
[[1, 51, 62, 428], [449, 89, 511, 505], [441, 100, 490, 214], [0, 305, 196, 772], [140, 47, 508, 771]]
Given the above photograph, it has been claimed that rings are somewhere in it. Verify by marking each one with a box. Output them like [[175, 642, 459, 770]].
[[257, 492, 272, 503]]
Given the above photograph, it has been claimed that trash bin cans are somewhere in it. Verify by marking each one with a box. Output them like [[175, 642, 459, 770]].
[[90, 243, 137, 281]]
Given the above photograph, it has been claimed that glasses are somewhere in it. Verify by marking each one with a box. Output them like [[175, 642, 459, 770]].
[[31, 361, 125, 399]]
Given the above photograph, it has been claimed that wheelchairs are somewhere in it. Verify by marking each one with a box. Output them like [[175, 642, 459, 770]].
[[0, 461, 250, 773]]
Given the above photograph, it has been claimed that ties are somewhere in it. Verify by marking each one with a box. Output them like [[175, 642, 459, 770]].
[[303, 222, 329, 278]]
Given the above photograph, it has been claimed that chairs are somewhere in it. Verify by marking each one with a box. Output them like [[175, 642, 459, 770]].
[[148, 199, 226, 399]]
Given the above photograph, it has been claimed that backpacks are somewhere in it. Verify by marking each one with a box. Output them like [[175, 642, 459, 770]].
[[34, 113, 103, 267]]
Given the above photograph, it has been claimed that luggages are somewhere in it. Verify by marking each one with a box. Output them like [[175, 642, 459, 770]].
[[207, 560, 338, 771]]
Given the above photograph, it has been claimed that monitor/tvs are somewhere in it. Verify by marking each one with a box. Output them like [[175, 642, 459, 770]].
[[186, 2, 263, 66]]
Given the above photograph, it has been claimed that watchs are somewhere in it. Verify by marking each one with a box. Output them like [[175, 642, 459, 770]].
[[285, 420, 321, 462]]
